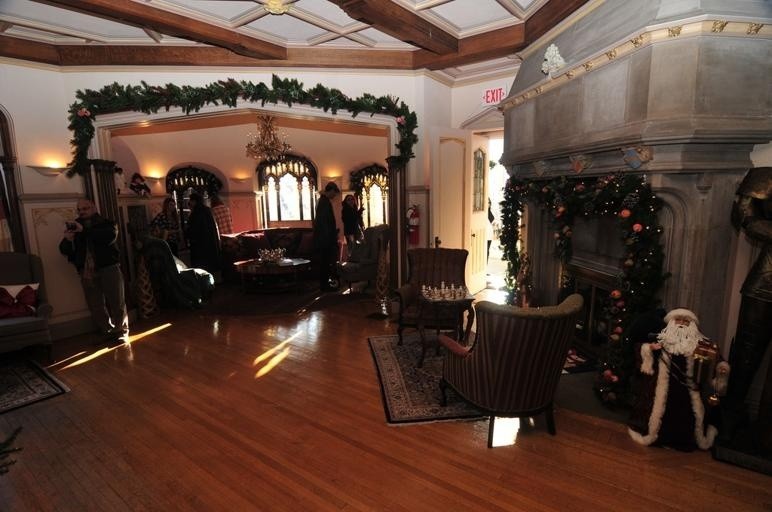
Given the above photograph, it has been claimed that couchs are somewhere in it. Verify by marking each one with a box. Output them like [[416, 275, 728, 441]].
[[339, 226, 389, 297], [0, 250, 57, 364], [222, 227, 315, 278]]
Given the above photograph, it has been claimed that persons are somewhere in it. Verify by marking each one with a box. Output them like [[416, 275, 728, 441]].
[[314, 182, 337, 291], [629, 307, 727, 452], [208, 196, 234, 283], [154, 198, 181, 259], [341, 196, 365, 259], [185, 192, 218, 272], [59, 198, 129, 345]]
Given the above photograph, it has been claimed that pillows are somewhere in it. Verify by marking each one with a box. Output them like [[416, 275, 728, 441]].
[[0, 282, 38, 315]]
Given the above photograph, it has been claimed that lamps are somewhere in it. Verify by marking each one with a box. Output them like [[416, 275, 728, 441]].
[[231, 171, 249, 183], [26, 160, 70, 177], [142, 167, 164, 184], [324, 170, 341, 183], [246, 113, 291, 166]]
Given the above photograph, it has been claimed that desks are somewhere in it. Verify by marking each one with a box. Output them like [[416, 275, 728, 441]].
[[411, 294, 475, 366]]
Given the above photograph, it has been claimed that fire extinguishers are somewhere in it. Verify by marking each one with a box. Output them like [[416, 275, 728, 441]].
[[404, 203, 420, 245]]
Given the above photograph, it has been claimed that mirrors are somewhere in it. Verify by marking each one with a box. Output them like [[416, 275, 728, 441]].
[[474, 148, 484, 209]]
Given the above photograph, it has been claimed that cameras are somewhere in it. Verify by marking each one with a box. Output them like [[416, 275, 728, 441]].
[[65, 223, 77, 232]]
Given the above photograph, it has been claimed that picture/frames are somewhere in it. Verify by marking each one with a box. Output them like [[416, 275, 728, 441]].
[[407, 185, 432, 250]]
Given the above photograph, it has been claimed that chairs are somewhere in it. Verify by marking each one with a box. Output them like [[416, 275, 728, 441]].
[[441, 291, 583, 450], [141, 238, 216, 311], [394, 246, 475, 369]]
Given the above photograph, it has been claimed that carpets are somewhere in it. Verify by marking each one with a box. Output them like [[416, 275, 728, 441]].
[[2, 353, 73, 412], [367, 330, 492, 426]]
